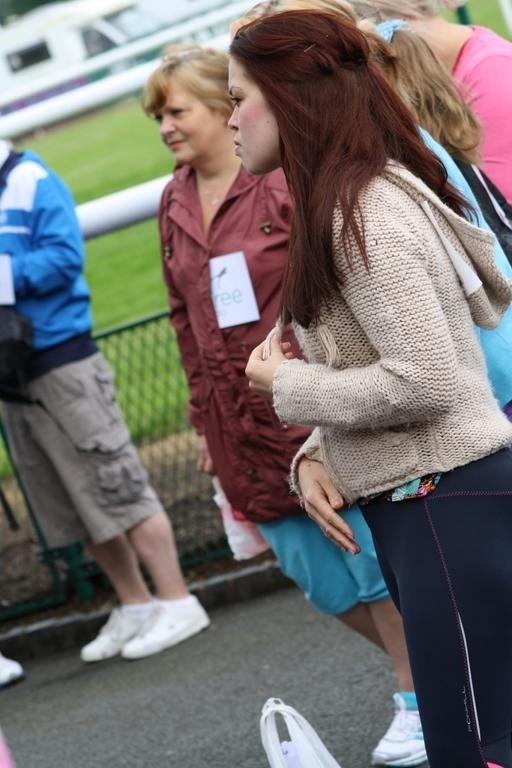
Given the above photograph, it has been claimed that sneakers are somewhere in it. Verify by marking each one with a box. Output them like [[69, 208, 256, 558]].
[[81, 599, 158, 662], [0, 652, 23, 686], [122, 596, 210, 660], [371, 692, 427, 766]]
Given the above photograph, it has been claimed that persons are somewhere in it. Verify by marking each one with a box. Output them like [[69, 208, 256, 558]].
[[0, 143, 209, 663], [142, 0, 511, 767]]
